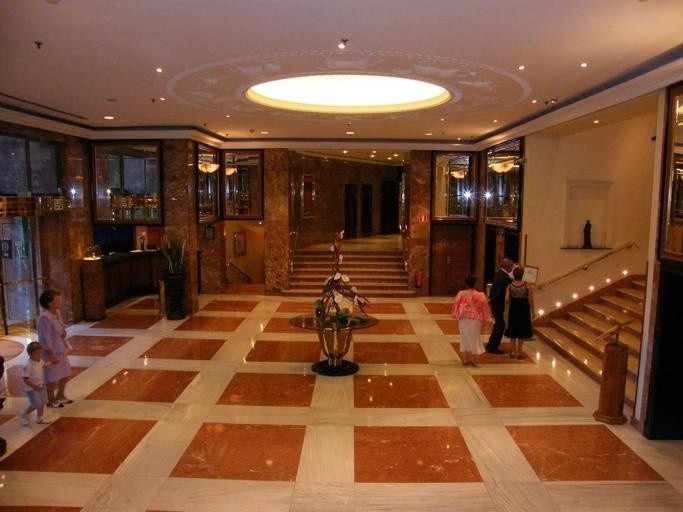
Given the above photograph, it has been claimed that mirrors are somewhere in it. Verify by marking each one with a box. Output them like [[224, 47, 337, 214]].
[[222, 149, 265, 221], [89, 139, 163, 227], [430, 149, 479, 226], [195, 142, 221, 226], [483, 137, 524, 232]]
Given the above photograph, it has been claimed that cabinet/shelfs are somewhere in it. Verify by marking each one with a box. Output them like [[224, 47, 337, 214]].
[[103, 251, 160, 309]]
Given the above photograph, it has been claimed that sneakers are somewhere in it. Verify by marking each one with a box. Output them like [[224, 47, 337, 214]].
[[462, 361, 478, 367], [19, 411, 30, 426], [508, 351, 525, 360], [32, 416, 51, 424], [46, 401, 64, 408], [54, 397, 74, 404]]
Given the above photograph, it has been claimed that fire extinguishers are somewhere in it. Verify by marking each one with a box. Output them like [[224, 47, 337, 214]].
[[415, 268, 424, 288]]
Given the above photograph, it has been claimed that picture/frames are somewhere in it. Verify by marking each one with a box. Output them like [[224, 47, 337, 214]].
[[232, 229, 248, 258]]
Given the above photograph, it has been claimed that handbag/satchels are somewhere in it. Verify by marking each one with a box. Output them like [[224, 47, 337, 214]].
[[64, 334, 74, 352]]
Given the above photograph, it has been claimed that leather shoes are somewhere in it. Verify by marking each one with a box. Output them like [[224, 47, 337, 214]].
[[485, 345, 505, 355]]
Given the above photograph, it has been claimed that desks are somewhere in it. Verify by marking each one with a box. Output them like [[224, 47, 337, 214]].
[[288, 312, 379, 377]]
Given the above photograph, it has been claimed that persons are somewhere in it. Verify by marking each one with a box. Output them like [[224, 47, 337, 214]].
[[452, 274, 490, 367], [37, 289, 74, 408], [486, 257, 515, 354], [506, 266, 535, 359], [20, 342, 59, 427]]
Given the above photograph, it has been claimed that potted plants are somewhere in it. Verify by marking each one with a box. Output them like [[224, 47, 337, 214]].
[[156, 233, 188, 322]]
